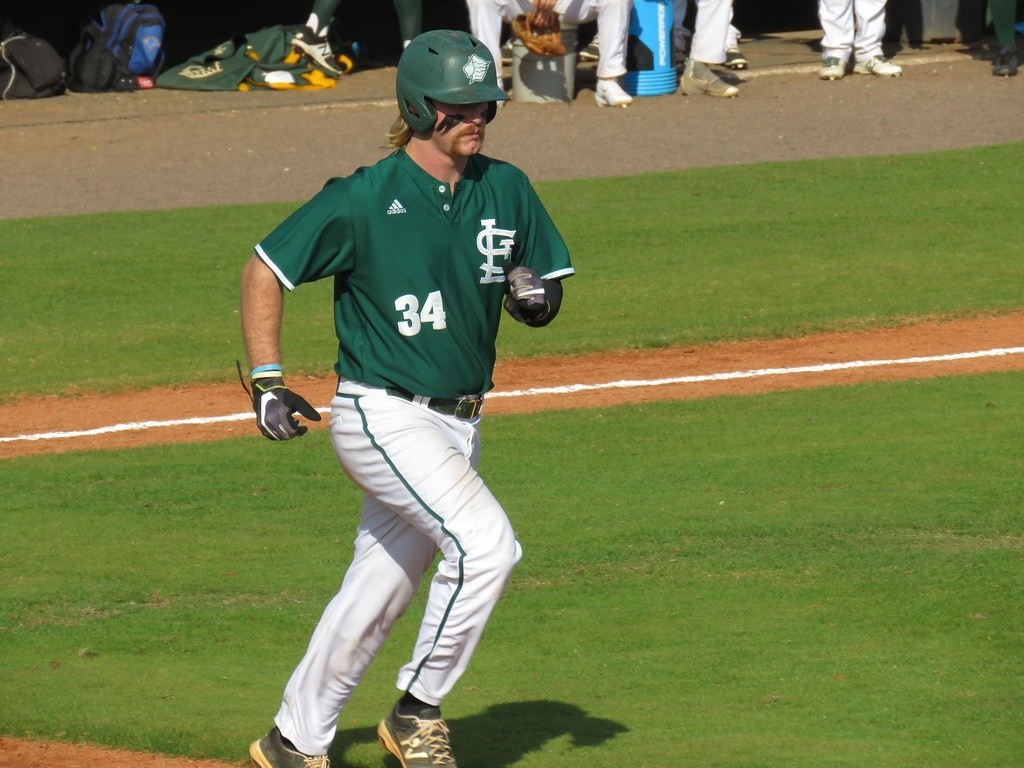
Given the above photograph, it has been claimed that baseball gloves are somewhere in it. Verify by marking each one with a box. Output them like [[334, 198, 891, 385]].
[[512, 7, 567, 57]]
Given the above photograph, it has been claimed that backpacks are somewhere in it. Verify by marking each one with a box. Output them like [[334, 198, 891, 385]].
[[0, 25, 63, 99], [67, 2, 167, 91]]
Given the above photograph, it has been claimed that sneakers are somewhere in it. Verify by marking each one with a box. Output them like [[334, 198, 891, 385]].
[[992, 45, 1020, 74], [853, 54, 903, 78], [724, 48, 748, 68], [579, 32, 599, 58], [292, 27, 343, 77], [249, 727, 331, 768], [377, 699, 457, 768], [594, 78, 633, 107], [818, 57, 846, 80], [679, 59, 739, 97]]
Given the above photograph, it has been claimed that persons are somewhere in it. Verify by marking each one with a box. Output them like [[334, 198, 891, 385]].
[[240, 30, 576, 768], [465, 0, 634, 108], [990, 0, 1018, 76], [817, 0, 902, 79], [291, 0, 422, 79], [673, 0, 748, 98]]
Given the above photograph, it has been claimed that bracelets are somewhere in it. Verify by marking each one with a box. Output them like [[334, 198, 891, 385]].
[[251, 364, 282, 379]]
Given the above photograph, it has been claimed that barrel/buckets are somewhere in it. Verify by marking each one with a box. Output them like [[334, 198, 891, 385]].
[[510, 23, 578, 102]]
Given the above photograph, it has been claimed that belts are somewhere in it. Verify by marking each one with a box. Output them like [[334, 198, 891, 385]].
[[385, 386, 483, 420]]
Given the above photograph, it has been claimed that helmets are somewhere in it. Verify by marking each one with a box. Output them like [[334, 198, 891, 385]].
[[395, 30, 510, 134]]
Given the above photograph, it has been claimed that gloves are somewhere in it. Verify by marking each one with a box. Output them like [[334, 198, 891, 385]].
[[502, 261, 546, 318], [251, 371, 321, 441]]
